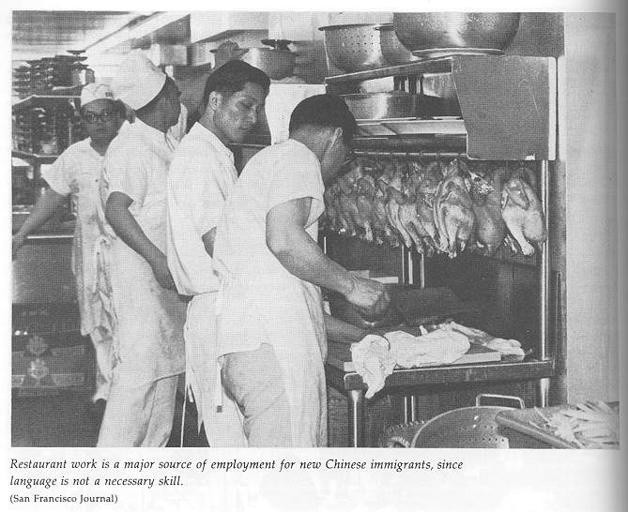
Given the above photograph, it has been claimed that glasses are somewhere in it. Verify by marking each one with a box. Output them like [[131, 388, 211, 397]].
[[82, 110, 117, 124]]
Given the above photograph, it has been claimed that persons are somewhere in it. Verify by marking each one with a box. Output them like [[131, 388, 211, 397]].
[[105, 39, 249, 445], [212, 93, 393, 446], [172, 59, 271, 445], [12, 80, 129, 403]]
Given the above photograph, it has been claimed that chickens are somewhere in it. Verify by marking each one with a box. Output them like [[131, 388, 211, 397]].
[[322, 156, 548, 259]]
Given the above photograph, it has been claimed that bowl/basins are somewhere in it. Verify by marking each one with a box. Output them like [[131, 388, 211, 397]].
[[354, 115, 466, 136], [391, 12, 522, 55], [324, 283, 417, 330], [210, 48, 298, 80]]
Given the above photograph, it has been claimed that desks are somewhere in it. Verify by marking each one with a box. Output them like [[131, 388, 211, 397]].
[[320, 363, 557, 448]]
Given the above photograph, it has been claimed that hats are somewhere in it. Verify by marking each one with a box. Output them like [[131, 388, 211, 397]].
[[80, 83, 117, 109], [113, 50, 167, 111]]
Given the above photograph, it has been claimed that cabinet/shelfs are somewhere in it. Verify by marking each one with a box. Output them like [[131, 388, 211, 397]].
[[325, 49, 560, 169], [12, 94, 83, 162], [230, 80, 328, 152]]
